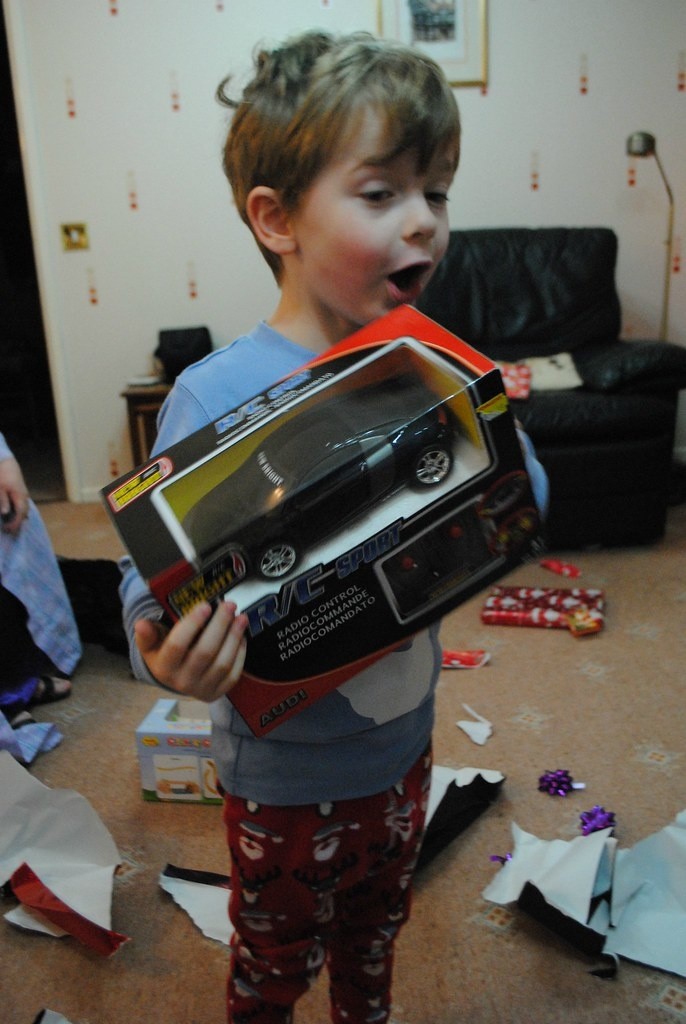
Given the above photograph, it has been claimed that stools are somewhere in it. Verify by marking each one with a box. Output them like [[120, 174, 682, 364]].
[[119, 367, 174, 471]]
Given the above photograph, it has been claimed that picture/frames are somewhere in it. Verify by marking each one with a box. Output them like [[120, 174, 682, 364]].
[[376, 0, 488, 93]]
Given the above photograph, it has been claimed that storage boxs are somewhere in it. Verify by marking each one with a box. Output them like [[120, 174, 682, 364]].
[[98, 301, 556, 740]]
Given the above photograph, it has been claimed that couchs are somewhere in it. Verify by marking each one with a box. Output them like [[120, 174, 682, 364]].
[[416, 225, 686, 557]]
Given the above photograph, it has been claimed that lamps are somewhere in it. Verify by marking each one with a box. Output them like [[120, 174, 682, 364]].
[[626, 132, 674, 342]]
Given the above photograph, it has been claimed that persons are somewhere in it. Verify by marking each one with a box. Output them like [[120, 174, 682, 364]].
[[118, 33, 549, 1023], [0, 433, 78, 733]]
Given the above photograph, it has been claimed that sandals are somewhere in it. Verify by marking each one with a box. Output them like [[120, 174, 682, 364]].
[[1, 675, 71, 713]]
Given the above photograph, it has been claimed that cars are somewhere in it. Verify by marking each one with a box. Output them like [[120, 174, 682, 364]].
[[178, 374, 456, 587]]
